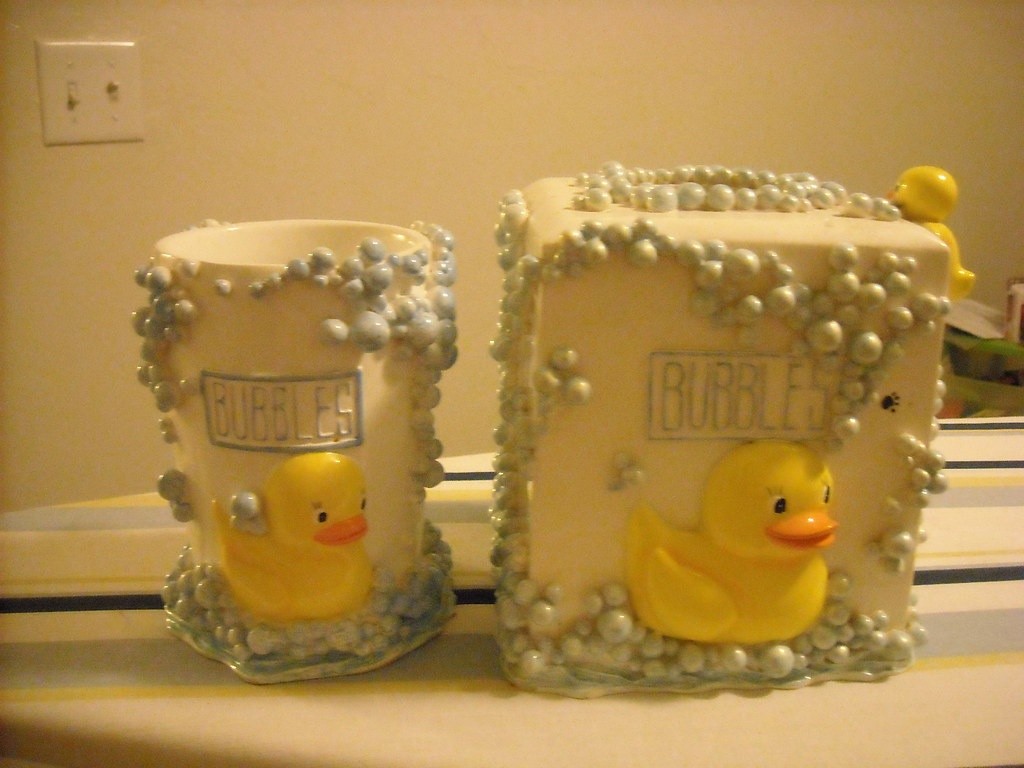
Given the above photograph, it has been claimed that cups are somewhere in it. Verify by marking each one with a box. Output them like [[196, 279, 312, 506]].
[[145, 221, 459, 685]]
